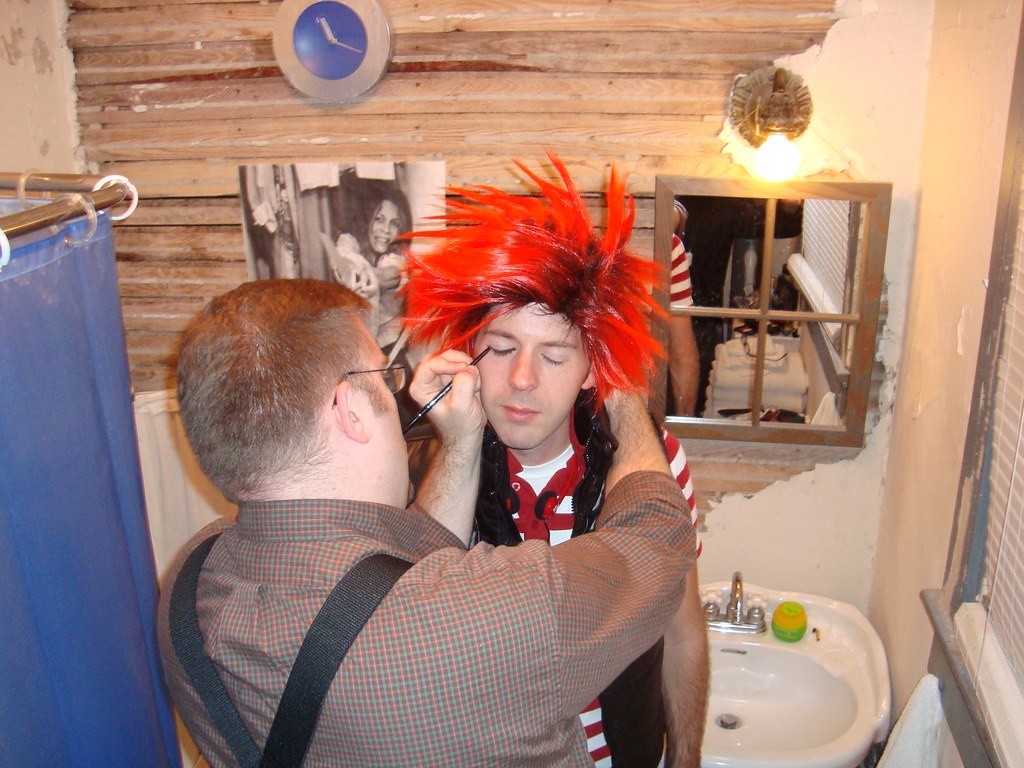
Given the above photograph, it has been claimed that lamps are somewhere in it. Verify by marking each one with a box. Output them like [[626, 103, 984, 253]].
[[731, 65, 813, 149]]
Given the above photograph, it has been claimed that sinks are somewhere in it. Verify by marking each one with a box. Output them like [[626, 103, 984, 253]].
[[701, 637, 891, 768]]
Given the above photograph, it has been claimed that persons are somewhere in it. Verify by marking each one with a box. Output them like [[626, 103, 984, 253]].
[[156, 279, 696, 768], [667, 198, 803, 414], [395, 153, 709, 768], [335, 185, 413, 336]]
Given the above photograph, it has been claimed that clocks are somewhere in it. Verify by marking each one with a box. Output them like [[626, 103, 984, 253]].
[[272, 0, 392, 104]]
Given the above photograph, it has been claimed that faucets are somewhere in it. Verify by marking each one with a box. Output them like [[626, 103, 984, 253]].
[[726, 571, 744, 626]]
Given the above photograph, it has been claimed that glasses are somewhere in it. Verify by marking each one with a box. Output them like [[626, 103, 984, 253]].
[[332, 361, 407, 410]]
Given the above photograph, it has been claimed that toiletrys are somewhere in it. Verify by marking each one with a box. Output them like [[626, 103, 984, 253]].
[[743, 237, 758, 288]]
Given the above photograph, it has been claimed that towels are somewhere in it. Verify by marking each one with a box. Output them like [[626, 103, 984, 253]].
[[874, 672, 966, 768], [810, 392, 841, 425], [703, 334, 809, 422]]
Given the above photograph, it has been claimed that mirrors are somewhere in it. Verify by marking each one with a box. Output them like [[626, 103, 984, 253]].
[[648, 176, 893, 448]]
